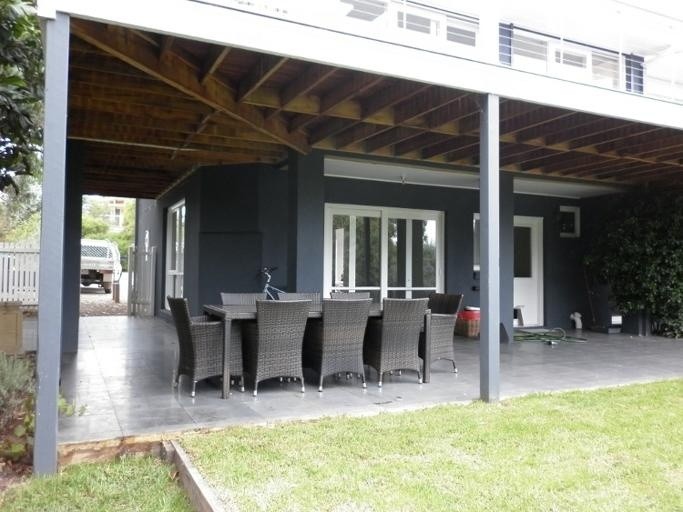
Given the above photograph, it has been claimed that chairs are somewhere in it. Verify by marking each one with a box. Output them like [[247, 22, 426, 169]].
[[167, 291, 464, 398]]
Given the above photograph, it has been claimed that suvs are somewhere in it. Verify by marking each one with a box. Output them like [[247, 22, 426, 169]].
[[79, 238, 122, 293]]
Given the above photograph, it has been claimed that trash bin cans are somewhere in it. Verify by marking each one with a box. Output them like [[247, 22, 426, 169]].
[[0, 301, 23, 357]]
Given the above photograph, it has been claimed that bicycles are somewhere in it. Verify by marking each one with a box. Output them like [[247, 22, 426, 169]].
[[259, 266, 288, 300]]
